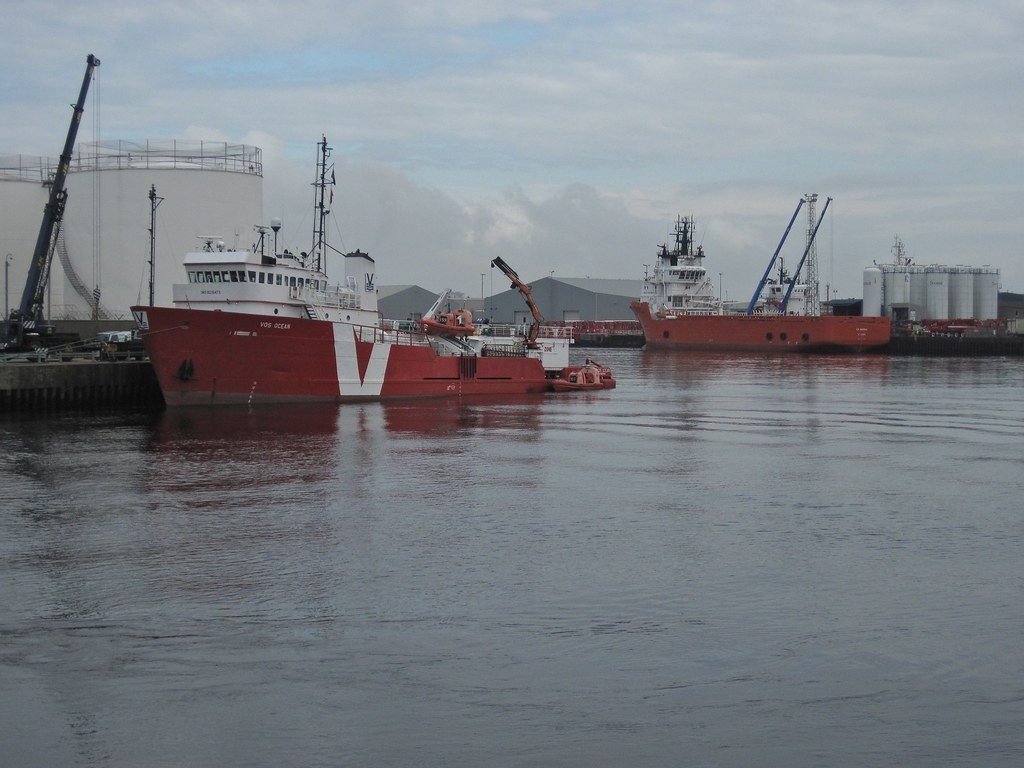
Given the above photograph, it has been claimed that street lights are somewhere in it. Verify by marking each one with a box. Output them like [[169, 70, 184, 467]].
[[481, 273, 485, 309]]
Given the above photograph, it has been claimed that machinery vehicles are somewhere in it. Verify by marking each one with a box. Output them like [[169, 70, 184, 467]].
[[1, 54, 102, 362]]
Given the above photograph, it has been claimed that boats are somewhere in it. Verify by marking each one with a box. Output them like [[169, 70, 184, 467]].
[[131, 133, 550, 409], [416, 309, 475, 336], [630, 213, 890, 351], [550, 357, 617, 390]]
